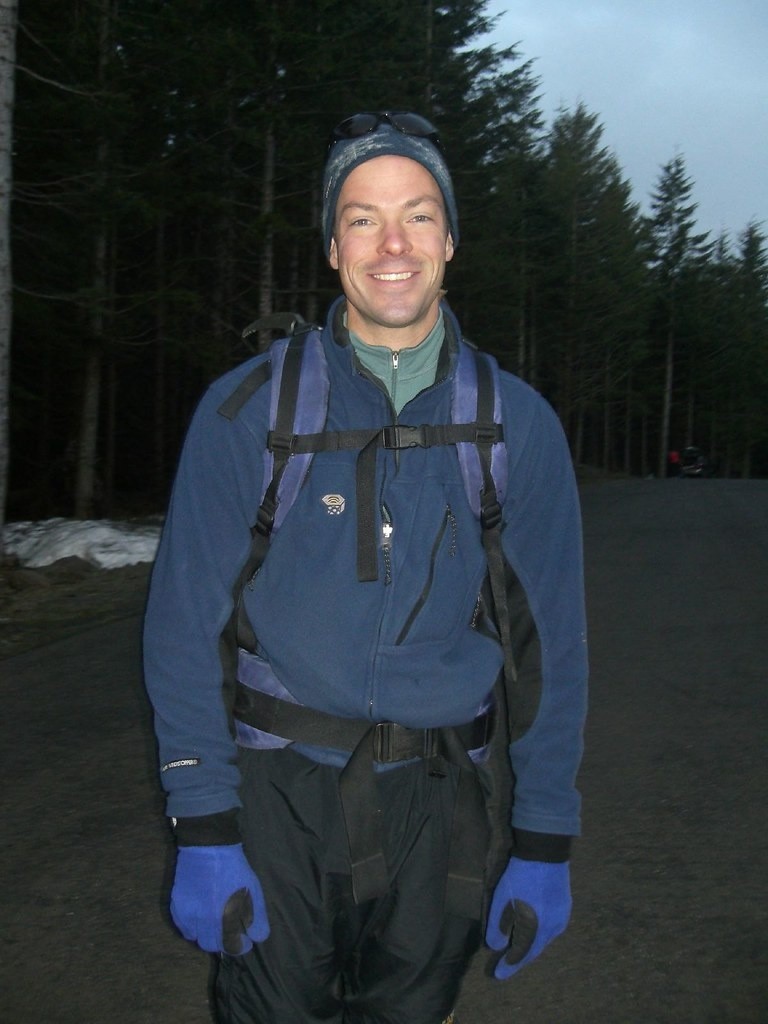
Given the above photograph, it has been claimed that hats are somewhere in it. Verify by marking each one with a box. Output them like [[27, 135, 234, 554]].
[[321, 122, 460, 262]]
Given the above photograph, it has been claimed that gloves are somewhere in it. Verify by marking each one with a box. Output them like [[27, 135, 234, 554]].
[[485, 825, 572, 981], [169, 809, 270, 957]]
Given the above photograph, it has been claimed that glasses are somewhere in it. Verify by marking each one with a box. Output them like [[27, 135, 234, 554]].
[[322, 111, 453, 164]]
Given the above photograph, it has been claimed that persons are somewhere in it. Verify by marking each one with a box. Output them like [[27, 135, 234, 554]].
[[146, 111, 590, 1024]]
